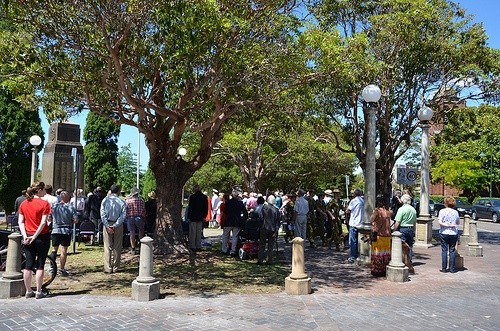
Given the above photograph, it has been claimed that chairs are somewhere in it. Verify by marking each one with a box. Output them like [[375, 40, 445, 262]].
[[7, 215, 19, 232], [78, 220, 96, 245], [239, 219, 260, 240], [98, 222, 103, 243]]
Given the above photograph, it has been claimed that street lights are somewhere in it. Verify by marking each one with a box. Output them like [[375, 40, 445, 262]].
[[479, 147, 493, 198], [28, 134, 42, 186], [412, 106, 435, 247], [356, 83, 383, 266]]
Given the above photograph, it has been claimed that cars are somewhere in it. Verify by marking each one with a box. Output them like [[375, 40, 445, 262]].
[[472, 198, 500, 224], [433, 197, 471, 218], [413, 197, 438, 212]]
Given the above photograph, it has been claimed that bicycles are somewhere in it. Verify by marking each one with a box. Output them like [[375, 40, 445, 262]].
[[0, 236, 58, 291]]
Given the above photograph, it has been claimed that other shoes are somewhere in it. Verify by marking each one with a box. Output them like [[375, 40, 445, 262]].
[[50, 268, 56, 276], [59, 269, 69, 277], [36, 292, 48, 300], [440, 268, 447, 272], [131, 249, 137, 255], [26, 292, 36, 298], [335, 245, 344, 252], [450, 269, 458, 273], [310, 242, 317, 248]]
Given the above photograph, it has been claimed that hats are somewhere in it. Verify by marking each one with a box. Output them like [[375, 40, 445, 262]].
[[129, 188, 141, 197], [331, 189, 341, 193], [213, 188, 218, 193]]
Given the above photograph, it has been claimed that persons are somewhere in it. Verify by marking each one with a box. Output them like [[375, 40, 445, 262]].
[[292, 189, 310, 253], [391, 190, 402, 219], [438, 195, 460, 273], [369, 196, 390, 278], [322, 189, 333, 205], [314, 193, 327, 247], [185, 188, 310, 243], [189, 184, 208, 252], [341, 188, 365, 263], [124, 187, 148, 255], [326, 188, 347, 252], [256, 193, 281, 265], [393, 194, 416, 275], [47, 191, 78, 276], [303, 190, 318, 248], [220, 190, 249, 256], [17, 182, 51, 299], [4, 185, 157, 240], [100, 184, 127, 274], [402, 188, 415, 208]]
